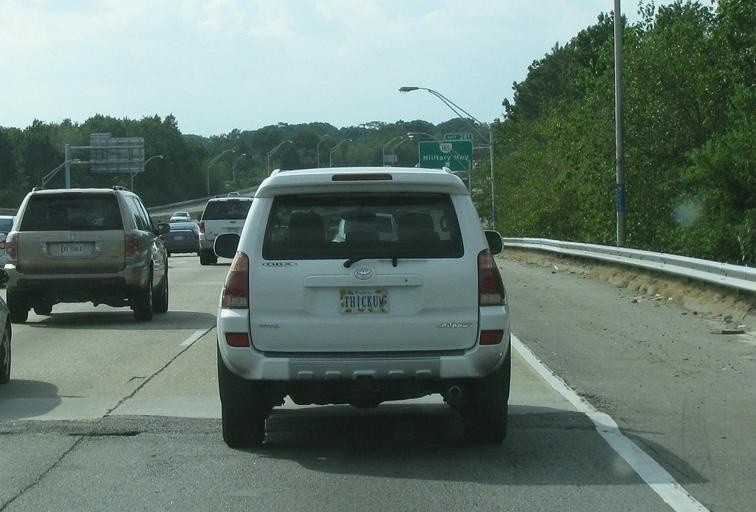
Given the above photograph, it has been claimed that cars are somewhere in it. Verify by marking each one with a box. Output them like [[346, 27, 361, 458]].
[[161, 222, 200, 256], [0, 294, 12, 383], [0, 215, 19, 281], [329, 211, 400, 243], [225, 191, 241, 197], [168, 210, 192, 223]]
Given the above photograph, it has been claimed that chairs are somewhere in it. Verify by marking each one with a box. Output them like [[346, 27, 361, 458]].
[[286, 213, 325, 251], [395, 212, 441, 257]]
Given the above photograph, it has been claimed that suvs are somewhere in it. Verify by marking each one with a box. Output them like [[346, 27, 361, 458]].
[[4, 182, 173, 323], [211, 164, 516, 449]]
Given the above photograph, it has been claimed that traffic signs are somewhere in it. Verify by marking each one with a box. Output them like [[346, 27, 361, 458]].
[[417, 140, 473, 175]]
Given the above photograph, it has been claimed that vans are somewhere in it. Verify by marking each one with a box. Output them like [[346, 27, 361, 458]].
[[198, 194, 255, 267]]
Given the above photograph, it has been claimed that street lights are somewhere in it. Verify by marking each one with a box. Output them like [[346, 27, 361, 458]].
[[267, 139, 295, 175], [231, 153, 248, 184], [315, 133, 330, 167], [129, 154, 164, 191], [205, 149, 235, 197], [328, 138, 353, 165], [382, 136, 412, 165], [397, 85, 499, 231], [40, 157, 83, 187], [442, 131, 474, 140], [406, 132, 472, 200]]
